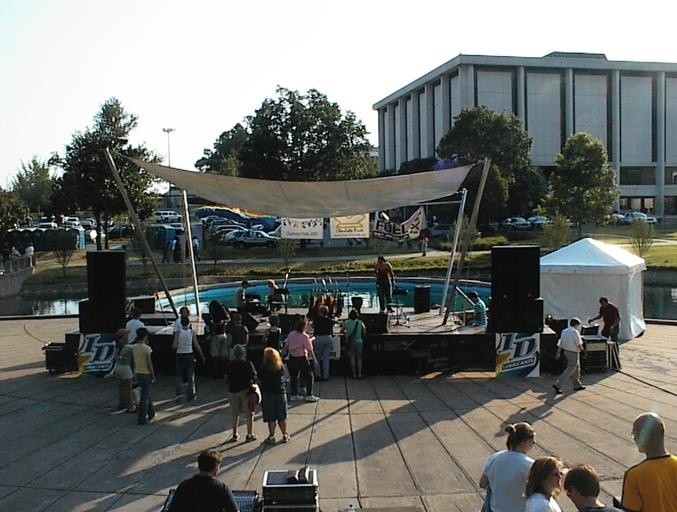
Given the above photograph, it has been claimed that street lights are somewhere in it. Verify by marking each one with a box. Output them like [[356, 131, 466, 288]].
[[159, 126, 176, 207]]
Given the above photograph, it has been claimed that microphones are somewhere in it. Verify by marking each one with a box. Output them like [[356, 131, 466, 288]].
[[346, 261, 350, 268], [285, 268, 291, 279]]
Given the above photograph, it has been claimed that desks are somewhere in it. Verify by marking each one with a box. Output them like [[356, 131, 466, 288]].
[[248, 344, 267, 361]]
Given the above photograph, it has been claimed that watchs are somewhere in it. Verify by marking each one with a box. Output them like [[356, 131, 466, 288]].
[[610, 326, 614, 329]]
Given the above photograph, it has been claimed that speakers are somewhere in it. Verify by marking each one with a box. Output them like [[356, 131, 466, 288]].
[[228, 310, 260, 332], [359, 313, 390, 332], [491, 245, 539, 304], [414, 285, 431, 313], [80, 251, 127, 333], [487, 300, 543, 332], [275, 313, 309, 333], [549, 316, 568, 334], [132, 297, 156, 313]]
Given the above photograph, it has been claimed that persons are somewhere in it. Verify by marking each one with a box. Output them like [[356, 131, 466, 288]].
[[478, 421, 536, 512], [565, 462, 623, 512], [523, 456, 565, 512], [167, 448, 241, 511], [374, 255, 396, 313], [466, 290, 487, 326], [421, 234, 429, 256], [24, 241, 34, 258], [159, 234, 201, 264], [107, 279, 369, 445], [588, 296, 621, 356], [551, 317, 586, 394], [11, 246, 21, 272], [620, 411, 677, 512]]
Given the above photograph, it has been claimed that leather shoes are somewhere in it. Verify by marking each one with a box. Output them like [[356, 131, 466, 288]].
[[551, 384, 585, 394]]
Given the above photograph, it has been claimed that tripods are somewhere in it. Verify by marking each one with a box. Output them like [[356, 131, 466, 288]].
[[388, 297, 409, 328]]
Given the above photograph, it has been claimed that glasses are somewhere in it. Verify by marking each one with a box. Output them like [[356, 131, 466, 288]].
[[548, 472, 562, 477]]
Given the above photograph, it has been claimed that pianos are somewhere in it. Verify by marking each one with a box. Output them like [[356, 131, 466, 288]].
[[377, 289, 408, 297]]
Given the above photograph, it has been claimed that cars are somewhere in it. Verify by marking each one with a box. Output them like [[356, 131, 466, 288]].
[[146, 224, 177, 241], [502, 217, 531, 232], [528, 216, 554, 229], [200, 216, 292, 249], [605, 210, 659, 226], [14, 213, 137, 243], [169, 222, 185, 234]]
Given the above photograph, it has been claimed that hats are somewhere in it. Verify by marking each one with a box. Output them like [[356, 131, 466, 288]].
[[115, 329, 129, 337], [241, 279, 251, 286]]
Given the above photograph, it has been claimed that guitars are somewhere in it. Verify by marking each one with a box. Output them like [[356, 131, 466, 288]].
[[308, 278, 344, 319]]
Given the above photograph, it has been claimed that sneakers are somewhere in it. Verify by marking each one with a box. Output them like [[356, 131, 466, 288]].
[[110, 377, 320, 445]]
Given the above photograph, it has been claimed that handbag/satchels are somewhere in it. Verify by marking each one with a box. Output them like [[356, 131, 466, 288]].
[[344, 336, 352, 346]]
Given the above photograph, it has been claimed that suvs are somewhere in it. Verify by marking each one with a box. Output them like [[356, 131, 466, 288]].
[[155, 210, 183, 223], [427, 224, 456, 241]]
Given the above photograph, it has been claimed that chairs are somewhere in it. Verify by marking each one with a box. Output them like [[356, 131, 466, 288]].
[[271, 286, 288, 316], [387, 288, 411, 329]]
